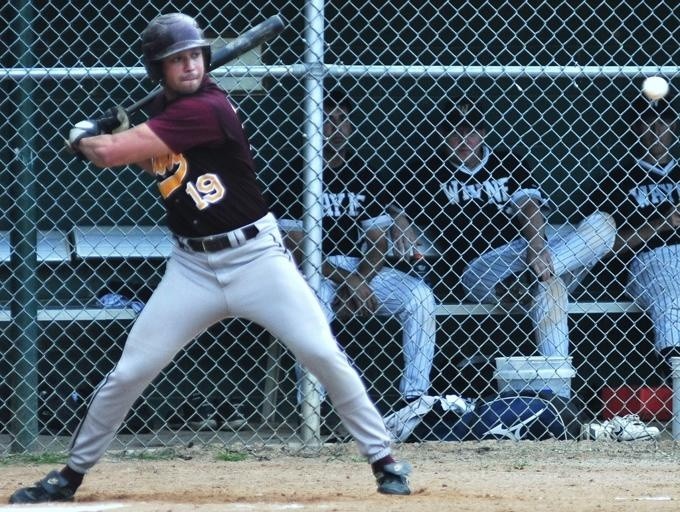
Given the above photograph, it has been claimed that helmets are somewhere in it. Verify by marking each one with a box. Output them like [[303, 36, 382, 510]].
[[141, 12, 211, 80]]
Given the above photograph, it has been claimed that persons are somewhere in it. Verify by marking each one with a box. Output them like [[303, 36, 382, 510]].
[[277, 91, 439, 443], [7, 10, 410, 506], [589, 95, 680, 382], [382, 93, 618, 396]]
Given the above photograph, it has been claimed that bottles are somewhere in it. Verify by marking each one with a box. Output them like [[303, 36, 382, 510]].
[[413, 252, 429, 286]]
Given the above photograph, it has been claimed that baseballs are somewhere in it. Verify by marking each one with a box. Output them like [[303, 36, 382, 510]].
[[644, 77, 669, 100]]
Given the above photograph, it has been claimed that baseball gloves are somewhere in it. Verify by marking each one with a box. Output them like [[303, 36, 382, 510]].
[[92, 16, 286, 128]]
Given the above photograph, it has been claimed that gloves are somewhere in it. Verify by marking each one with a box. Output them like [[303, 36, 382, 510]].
[[69, 105, 129, 161]]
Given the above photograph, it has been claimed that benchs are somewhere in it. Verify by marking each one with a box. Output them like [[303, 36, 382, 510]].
[[0, 215, 651, 428]]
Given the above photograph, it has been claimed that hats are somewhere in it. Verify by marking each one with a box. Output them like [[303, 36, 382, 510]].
[[634, 92, 676, 120], [441, 97, 484, 131]]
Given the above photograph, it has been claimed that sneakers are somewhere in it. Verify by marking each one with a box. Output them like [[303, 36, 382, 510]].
[[581, 413, 662, 444], [493, 267, 542, 305], [9, 469, 77, 504], [373, 462, 412, 495]]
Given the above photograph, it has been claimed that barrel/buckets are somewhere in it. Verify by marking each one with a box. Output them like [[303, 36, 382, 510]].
[[493, 356, 577, 398]]
